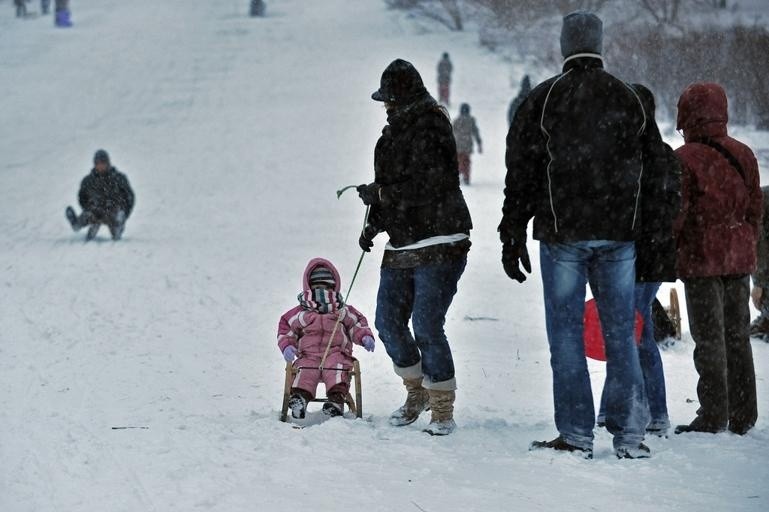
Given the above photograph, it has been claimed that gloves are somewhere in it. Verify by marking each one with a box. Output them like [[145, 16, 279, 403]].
[[500, 228, 532, 283], [362, 335, 375, 352], [283, 344, 298, 362], [359, 223, 381, 252], [356, 182, 383, 205]]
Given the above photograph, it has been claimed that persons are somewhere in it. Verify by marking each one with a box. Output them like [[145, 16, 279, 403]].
[[10, 0, 73, 28], [355, 58, 472, 436], [277, 258, 375, 419], [597, 85, 681, 433], [674, 82, 762, 437], [496, 12, 662, 458], [437, 53, 451, 105], [750, 186, 768, 339], [507, 75, 531, 124], [451, 105, 482, 186], [65, 150, 134, 239]]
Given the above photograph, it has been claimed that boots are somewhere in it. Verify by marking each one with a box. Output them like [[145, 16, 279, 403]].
[[389, 357, 430, 426], [322, 396, 344, 416], [288, 392, 307, 419], [421, 374, 457, 436]]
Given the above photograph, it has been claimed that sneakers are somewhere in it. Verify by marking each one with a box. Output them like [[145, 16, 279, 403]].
[[597, 412, 607, 428], [674, 421, 752, 436], [528, 434, 593, 458], [647, 424, 671, 439], [67, 207, 81, 231], [614, 439, 651, 459]]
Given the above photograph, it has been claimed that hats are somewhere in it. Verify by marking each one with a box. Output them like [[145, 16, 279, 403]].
[[93, 148, 109, 163], [311, 265, 336, 286], [370, 58, 428, 101], [560, 9, 604, 55]]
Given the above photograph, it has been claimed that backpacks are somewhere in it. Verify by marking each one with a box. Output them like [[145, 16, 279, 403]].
[[664, 144, 698, 232]]
